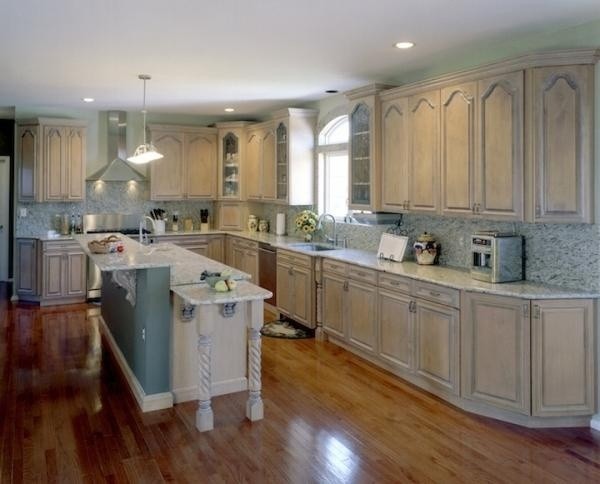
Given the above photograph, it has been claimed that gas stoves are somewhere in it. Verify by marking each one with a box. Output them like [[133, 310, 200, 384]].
[[88, 229, 151, 236]]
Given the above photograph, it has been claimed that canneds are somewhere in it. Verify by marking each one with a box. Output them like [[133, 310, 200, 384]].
[[259, 220, 269, 233]]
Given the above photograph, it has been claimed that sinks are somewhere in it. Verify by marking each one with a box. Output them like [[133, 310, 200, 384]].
[[287, 243, 340, 252]]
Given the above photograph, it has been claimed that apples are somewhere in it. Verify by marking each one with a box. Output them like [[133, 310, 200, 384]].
[[118, 246, 123, 252]]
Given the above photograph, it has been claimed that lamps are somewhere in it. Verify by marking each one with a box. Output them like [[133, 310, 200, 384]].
[[125, 74, 165, 168]]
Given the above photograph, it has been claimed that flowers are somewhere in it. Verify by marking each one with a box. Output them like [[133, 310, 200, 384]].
[[296, 209, 320, 234]]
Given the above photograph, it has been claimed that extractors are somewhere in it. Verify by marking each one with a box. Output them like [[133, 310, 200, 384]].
[[85, 110, 148, 183]]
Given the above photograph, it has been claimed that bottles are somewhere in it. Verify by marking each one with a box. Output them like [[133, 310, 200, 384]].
[[62, 213, 70, 235], [258, 219, 268, 232], [77, 215, 83, 234], [172, 214, 179, 231], [54, 214, 61, 233], [247, 214, 259, 233], [70, 215, 76, 235]]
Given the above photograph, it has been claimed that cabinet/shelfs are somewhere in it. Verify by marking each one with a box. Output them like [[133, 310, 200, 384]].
[[145, 106, 316, 207], [275, 241, 599, 429], [16, 232, 87, 308], [16, 115, 87, 204], [125, 227, 276, 315], [342, 48, 599, 227]]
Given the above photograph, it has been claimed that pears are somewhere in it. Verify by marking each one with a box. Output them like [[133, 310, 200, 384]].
[[214, 280, 228, 293], [222, 267, 233, 278], [227, 278, 238, 290]]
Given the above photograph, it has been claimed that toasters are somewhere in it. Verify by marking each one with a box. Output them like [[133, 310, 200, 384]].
[[468, 230, 524, 285]]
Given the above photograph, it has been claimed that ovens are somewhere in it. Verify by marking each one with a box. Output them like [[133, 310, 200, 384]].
[[86, 234, 151, 301]]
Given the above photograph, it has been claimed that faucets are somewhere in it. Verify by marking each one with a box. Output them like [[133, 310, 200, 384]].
[[317, 213, 337, 246]]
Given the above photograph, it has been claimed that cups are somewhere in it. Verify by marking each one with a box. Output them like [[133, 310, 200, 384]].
[[226, 152, 239, 161], [282, 175, 286, 183], [282, 133, 286, 141]]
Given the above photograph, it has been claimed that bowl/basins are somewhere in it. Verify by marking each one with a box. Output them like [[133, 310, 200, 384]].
[[205, 274, 228, 290]]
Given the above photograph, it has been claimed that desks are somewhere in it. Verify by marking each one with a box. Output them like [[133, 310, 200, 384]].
[[72, 234, 274, 432]]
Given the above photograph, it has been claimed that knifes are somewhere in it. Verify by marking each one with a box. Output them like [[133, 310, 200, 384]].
[[149, 208, 164, 221], [200, 208, 208, 223]]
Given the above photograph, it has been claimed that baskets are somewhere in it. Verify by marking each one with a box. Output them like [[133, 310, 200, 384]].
[[88, 235, 123, 254]]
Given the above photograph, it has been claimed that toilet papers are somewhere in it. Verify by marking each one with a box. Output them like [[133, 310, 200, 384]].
[[276, 212, 286, 235]]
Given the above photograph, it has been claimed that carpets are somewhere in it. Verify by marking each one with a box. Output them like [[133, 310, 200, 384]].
[[260, 318, 316, 340]]
